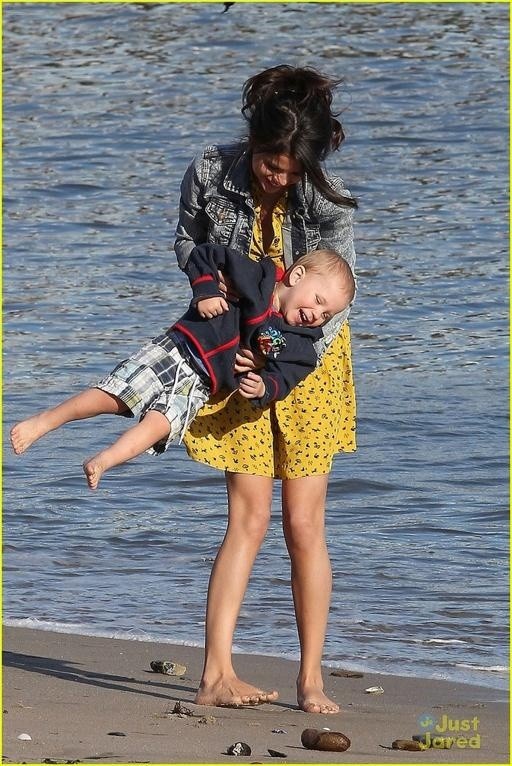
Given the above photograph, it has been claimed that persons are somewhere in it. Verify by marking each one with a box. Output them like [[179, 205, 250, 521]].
[[173, 63, 357, 714], [11, 249, 355, 491]]
